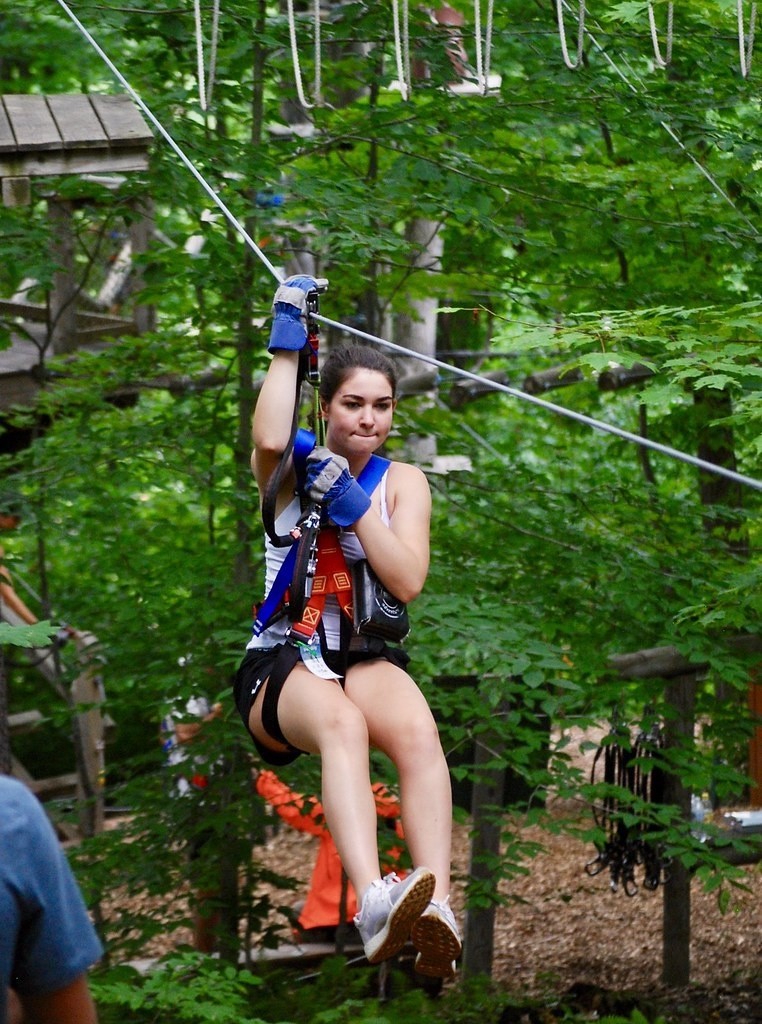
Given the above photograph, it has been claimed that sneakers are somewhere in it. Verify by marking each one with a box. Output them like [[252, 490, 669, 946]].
[[351, 865, 436, 964], [410, 895, 461, 978]]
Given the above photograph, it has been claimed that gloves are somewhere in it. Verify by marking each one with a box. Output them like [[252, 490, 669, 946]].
[[304, 446, 371, 526], [268, 275, 328, 353]]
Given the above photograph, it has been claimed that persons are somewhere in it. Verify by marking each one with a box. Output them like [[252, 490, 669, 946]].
[[232, 275, 462, 978], [0, 775, 105, 1024], [159, 639, 241, 967]]
